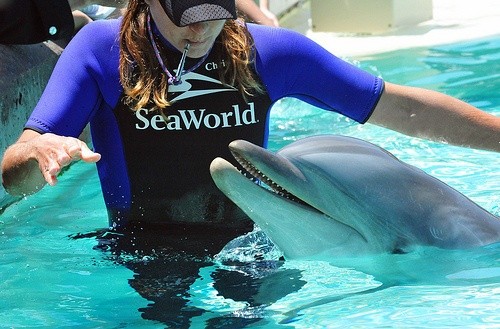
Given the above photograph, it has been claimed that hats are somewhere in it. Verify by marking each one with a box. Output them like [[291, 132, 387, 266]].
[[158, 0, 237, 27]]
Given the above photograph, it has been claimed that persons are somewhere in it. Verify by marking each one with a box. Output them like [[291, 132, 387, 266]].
[[0, 0, 500, 328], [0, 0, 131, 46]]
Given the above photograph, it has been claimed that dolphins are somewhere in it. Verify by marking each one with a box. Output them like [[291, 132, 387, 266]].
[[209, 135, 499, 261]]
